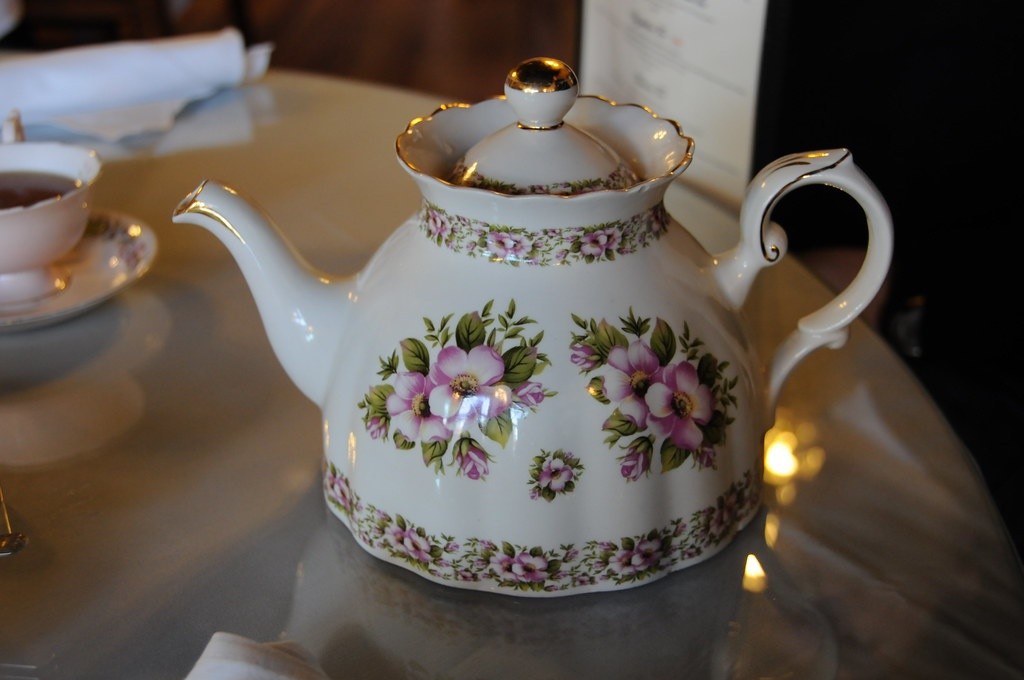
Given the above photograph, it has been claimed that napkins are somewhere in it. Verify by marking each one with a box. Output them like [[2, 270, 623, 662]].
[[0, 28, 273, 129]]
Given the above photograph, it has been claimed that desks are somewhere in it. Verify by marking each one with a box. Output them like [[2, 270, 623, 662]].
[[0, 51, 1024, 680]]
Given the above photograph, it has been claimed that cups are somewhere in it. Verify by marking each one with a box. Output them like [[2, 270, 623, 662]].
[[0, 115, 103, 307]]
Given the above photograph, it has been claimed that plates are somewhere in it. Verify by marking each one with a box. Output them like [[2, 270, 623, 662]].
[[0, 206, 158, 331]]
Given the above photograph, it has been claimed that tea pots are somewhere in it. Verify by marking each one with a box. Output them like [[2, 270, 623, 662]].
[[172, 56, 892, 597]]
